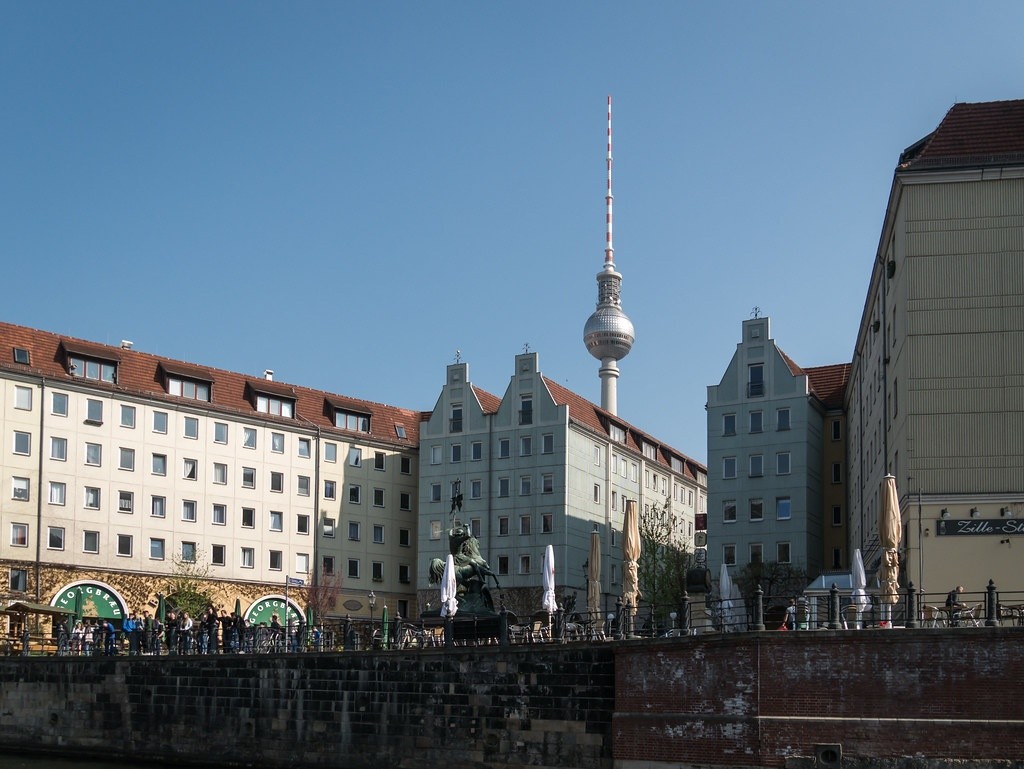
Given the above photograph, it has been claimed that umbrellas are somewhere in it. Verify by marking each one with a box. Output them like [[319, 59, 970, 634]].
[[439, 554, 459, 643], [623, 500, 641, 637], [156, 594, 166, 650], [851, 549, 872, 629], [875, 475, 903, 623], [542, 545, 558, 640], [720, 564, 747, 631], [307, 603, 313, 644], [382, 603, 389, 649], [589, 529, 601, 632], [236, 599, 241, 617]]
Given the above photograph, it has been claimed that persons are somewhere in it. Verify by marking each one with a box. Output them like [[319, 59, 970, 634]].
[[288, 618, 320, 651], [946, 586, 966, 627], [199, 605, 267, 654], [168, 612, 193, 654], [58, 617, 116, 656], [270, 616, 282, 652], [123, 611, 165, 656], [448, 524, 488, 584], [783, 600, 796, 630]]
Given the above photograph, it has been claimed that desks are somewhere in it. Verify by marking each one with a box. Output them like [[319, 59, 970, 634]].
[[937, 606, 969, 628], [1004, 605, 1024, 627], [513, 620, 594, 643]]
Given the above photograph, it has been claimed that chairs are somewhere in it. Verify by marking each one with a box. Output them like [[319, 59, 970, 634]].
[[996, 603, 1003, 626], [922, 605, 939, 628], [965, 603, 984, 627], [428, 619, 607, 647]]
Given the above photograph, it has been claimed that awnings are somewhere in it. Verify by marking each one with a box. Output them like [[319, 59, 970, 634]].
[[5, 603, 77, 626]]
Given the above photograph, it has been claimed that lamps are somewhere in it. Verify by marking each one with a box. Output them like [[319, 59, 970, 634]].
[[1001, 506, 1011, 516], [942, 508, 950, 519], [970, 507, 980, 519]]
